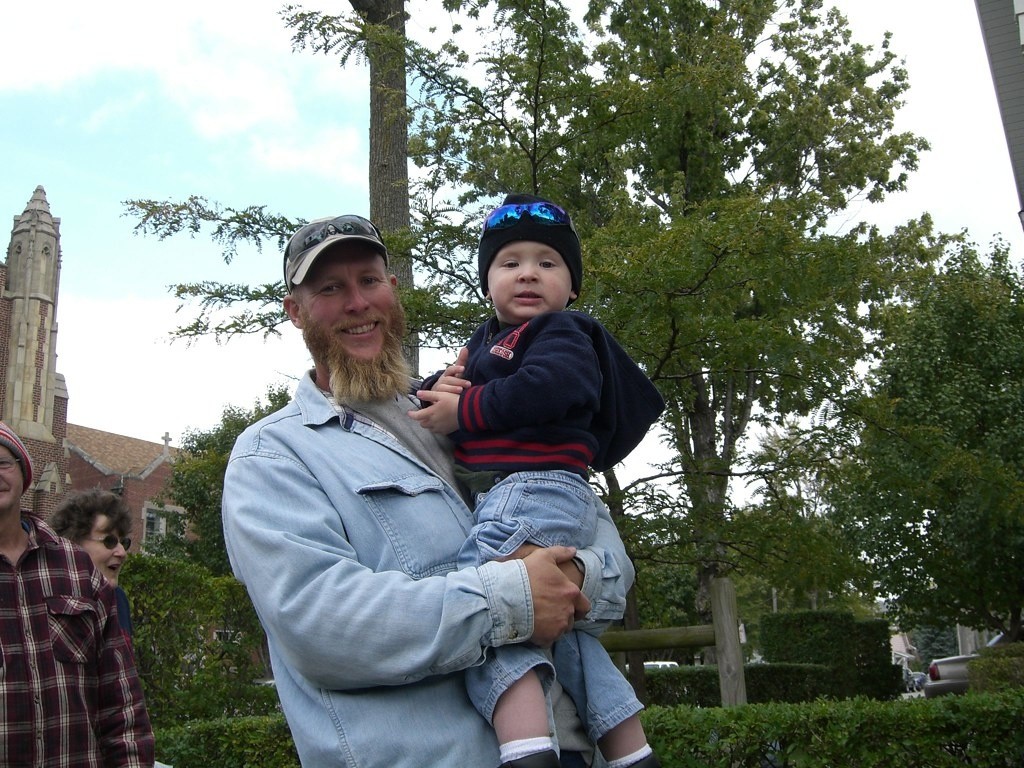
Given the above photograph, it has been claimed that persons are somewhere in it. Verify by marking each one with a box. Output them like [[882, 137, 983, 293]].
[[0, 420, 157, 768], [405, 193, 666, 768], [219, 213, 633, 768]]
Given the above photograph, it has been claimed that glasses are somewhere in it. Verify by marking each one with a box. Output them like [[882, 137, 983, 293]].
[[0, 458, 20, 473], [283, 215, 385, 295], [84, 534, 131, 552], [478, 201, 581, 250]]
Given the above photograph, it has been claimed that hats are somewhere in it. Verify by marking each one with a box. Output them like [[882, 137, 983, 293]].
[[480, 193, 584, 308], [284, 214, 390, 292], [0, 421, 34, 497]]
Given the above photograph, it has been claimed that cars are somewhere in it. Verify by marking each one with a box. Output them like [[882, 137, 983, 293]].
[[912, 671, 928, 692], [923, 634, 1004, 700], [900, 666, 917, 694]]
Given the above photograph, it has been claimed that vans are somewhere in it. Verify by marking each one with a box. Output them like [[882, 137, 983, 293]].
[[627, 660, 688, 697]]
